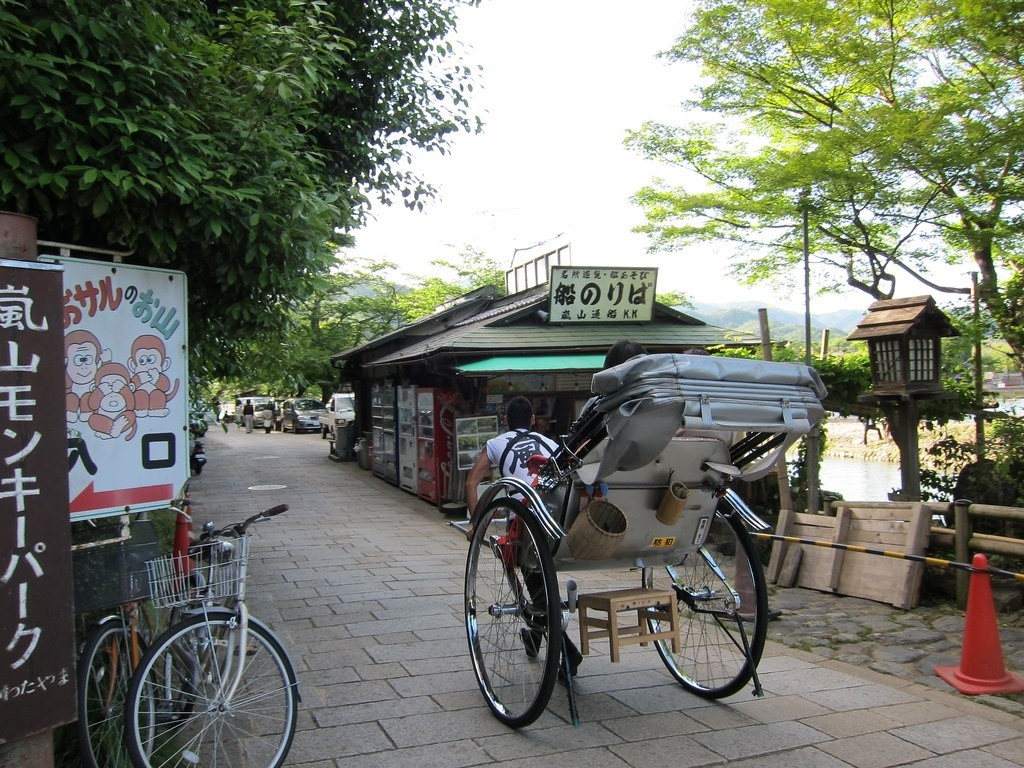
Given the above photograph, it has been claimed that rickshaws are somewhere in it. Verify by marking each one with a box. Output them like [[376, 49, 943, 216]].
[[447, 352, 830, 728]]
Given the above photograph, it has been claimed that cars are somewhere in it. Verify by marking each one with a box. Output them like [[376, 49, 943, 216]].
[[217, 387, 357, 440]]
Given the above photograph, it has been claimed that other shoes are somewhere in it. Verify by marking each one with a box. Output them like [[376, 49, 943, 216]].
[[557, 651, 584, 681], [519, 628, 539, 659]]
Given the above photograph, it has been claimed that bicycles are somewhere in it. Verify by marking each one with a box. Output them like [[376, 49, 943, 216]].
[[124, 503, 304, 768], [69, 498, 208, 768]]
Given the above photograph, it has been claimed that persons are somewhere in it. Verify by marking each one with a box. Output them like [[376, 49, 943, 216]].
[[489, 340, 649, 568], [234, 395, 277, 435], [465, 395, 585, 687]]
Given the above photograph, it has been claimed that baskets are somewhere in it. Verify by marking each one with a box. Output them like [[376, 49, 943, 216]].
[[144, 533, 254, 608], [71, 518, 160, 616]]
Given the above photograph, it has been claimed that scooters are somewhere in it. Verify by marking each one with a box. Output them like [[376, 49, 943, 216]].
[[190, 426, 207, 474]]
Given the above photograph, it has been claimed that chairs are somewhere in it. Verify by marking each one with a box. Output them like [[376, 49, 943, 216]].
[[505, 352, 813, 577]]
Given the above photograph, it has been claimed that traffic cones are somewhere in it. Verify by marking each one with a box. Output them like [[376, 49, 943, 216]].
[[934, 552, 1024, 696]]
